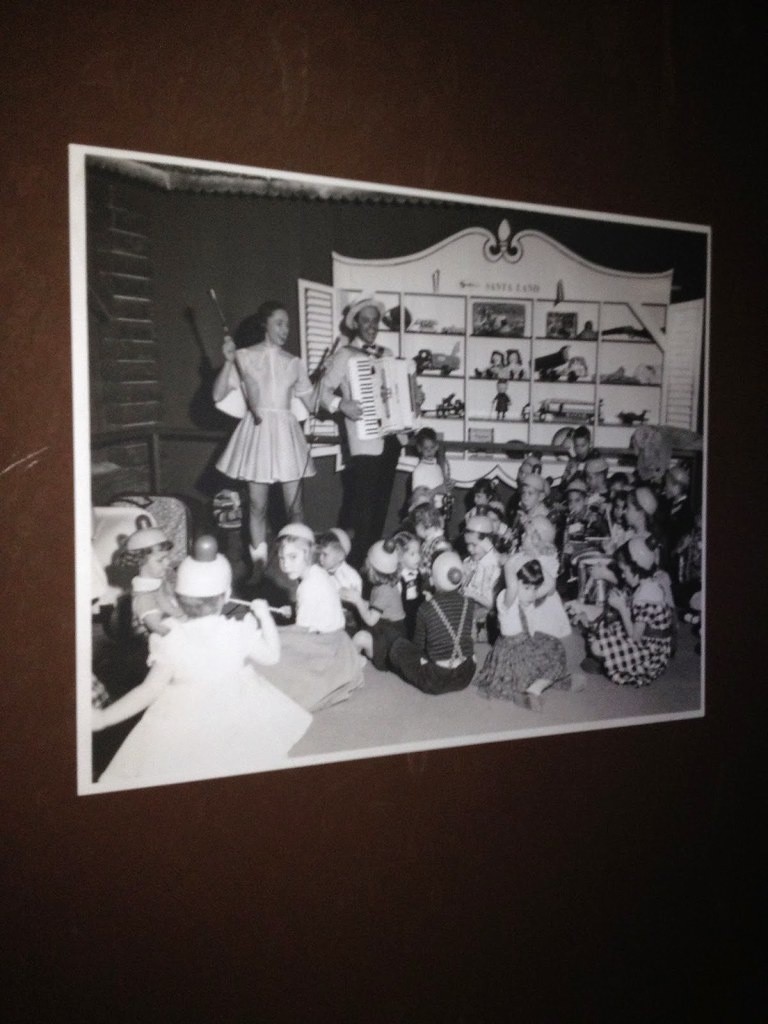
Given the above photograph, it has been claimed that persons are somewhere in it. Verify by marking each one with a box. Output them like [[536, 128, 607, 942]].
[[318, 296, 426, 569], [92, 424, 695, 783], [213, 299, 335, 586]]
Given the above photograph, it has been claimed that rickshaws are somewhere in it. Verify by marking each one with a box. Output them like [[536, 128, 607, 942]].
[[418, 393, 465, 418]]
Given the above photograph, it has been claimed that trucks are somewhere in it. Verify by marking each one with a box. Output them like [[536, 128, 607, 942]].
[[533, 344, 590, 383]]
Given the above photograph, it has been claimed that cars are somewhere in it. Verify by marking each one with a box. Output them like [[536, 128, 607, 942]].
[[413, 341, 464, 376]]
